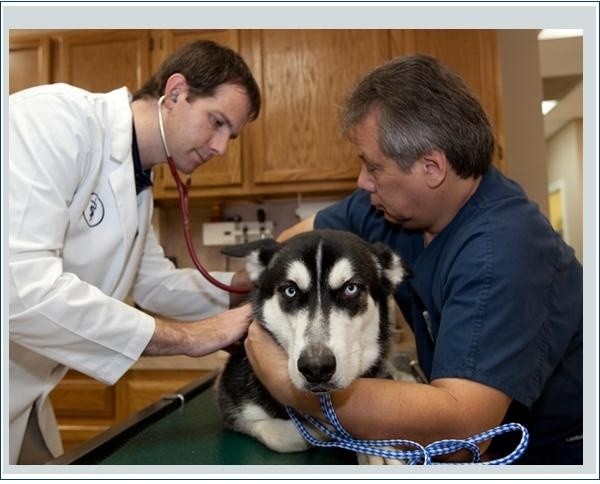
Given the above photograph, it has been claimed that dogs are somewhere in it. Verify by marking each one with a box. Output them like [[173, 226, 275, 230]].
[[212, 227, 417, 466]]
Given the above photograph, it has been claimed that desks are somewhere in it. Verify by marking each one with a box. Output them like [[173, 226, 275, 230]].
[[44, 366, 358, 464]]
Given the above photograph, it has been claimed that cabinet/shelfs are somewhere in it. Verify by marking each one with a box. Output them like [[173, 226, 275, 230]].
[[48, 369, 213, 452]]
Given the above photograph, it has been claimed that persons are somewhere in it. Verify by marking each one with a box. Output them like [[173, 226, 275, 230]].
[[276, 53, 582, 466], [7, 36, 261, 465]]
